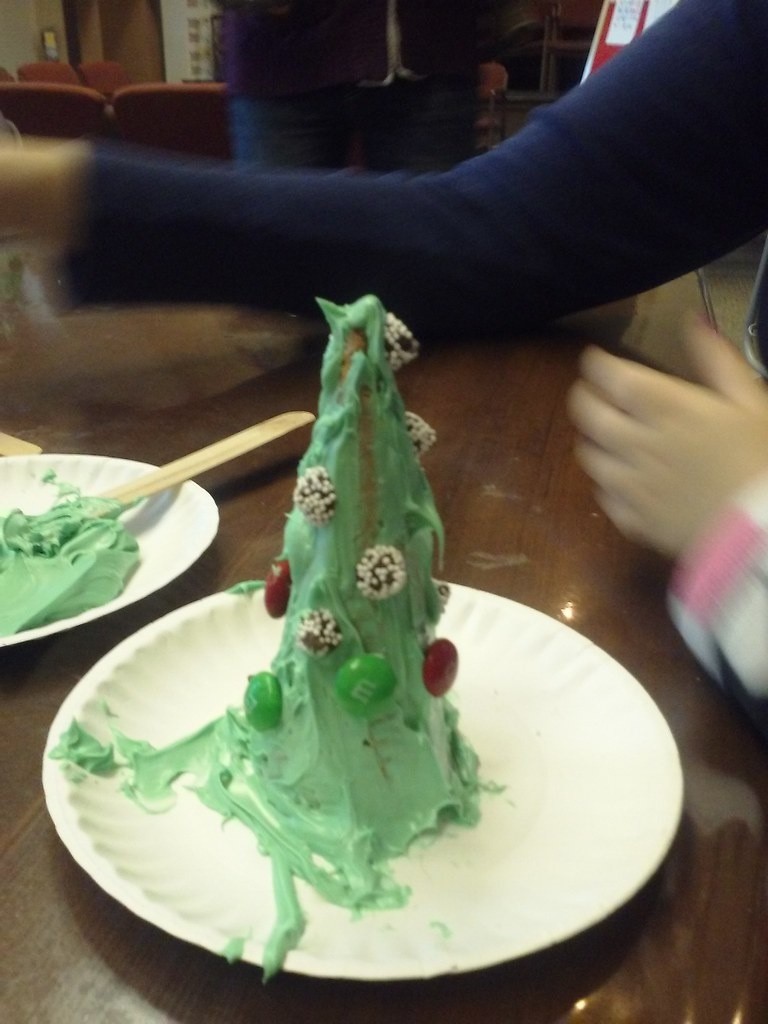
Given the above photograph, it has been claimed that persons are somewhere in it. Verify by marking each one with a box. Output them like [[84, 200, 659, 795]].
[[0, 0, 765, 384], [563, 305, 763, 735], [207, 0, 515, 200]]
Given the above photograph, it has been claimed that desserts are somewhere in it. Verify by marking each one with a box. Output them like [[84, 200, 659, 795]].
[[0, 292, 507, 986]]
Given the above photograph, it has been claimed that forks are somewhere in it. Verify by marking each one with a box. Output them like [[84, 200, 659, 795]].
[[24, 411, 315, 538]]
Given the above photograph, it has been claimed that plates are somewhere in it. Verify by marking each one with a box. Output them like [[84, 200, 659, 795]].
[[42, 580, 684, 982], [2, 450, 220, 646]]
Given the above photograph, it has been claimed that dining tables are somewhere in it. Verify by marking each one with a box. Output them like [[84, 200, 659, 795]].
[[1, 214, 766, 1020]]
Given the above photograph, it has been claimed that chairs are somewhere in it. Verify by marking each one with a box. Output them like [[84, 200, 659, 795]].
[[112, 78, 246, 170], [1, 79, 107, 165], [20, 61, 85, 93], [72, 59, 133, 97]]
